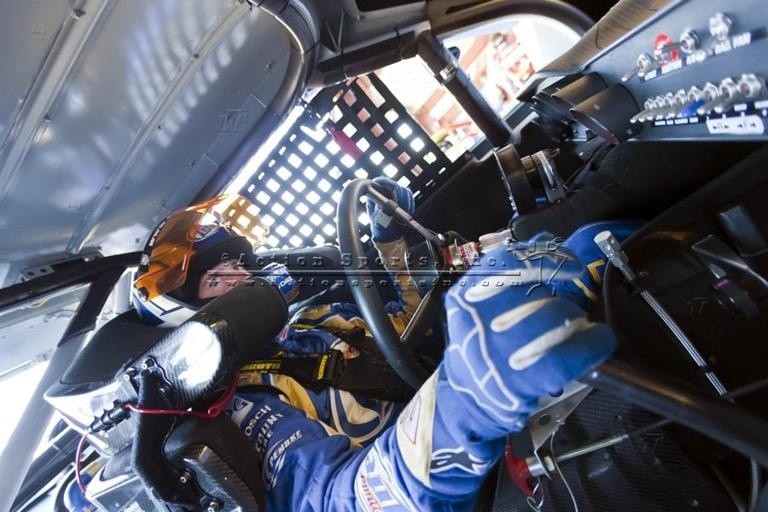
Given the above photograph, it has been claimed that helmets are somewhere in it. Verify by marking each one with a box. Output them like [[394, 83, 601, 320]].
[[131, 192, 290, 346]]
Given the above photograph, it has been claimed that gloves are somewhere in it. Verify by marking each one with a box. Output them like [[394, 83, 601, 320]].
[[395, 232, 619, 498], [366, 176, 416, 243]]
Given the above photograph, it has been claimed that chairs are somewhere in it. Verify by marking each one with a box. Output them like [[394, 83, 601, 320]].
[[42, 247, 673, 512]]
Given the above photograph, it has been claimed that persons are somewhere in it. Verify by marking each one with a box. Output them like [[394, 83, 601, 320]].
[[129, 172, 691, 511]]
